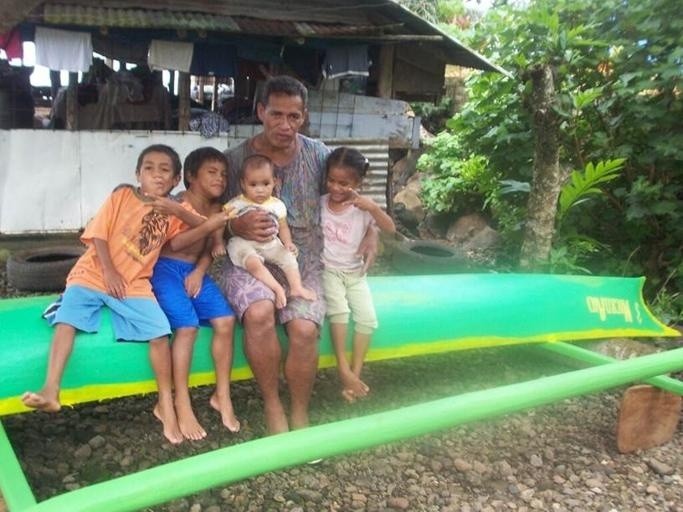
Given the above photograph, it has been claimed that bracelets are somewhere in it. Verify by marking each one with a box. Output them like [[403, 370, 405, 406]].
[[227, 218, 235, 238]]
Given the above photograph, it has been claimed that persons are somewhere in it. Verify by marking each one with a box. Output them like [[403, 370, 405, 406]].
[[318, 147, 395, 403], [21, 143, 209, 443], [207, 73, 379, 435], [150, 145, 241, 440], [211, 153, 317, 309]]
